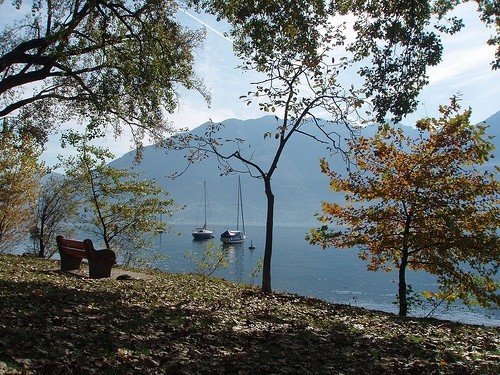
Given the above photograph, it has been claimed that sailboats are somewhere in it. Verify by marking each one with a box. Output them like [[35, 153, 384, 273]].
[[192, 179, 214, 239], [220, 174, 247, 243], [156, 200, 165, 233]]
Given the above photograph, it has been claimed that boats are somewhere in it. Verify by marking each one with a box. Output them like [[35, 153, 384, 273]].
[[249, 246, 255, 249]]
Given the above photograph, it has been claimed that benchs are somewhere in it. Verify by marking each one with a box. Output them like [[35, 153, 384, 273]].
[[56, 235, 117, 279]]
